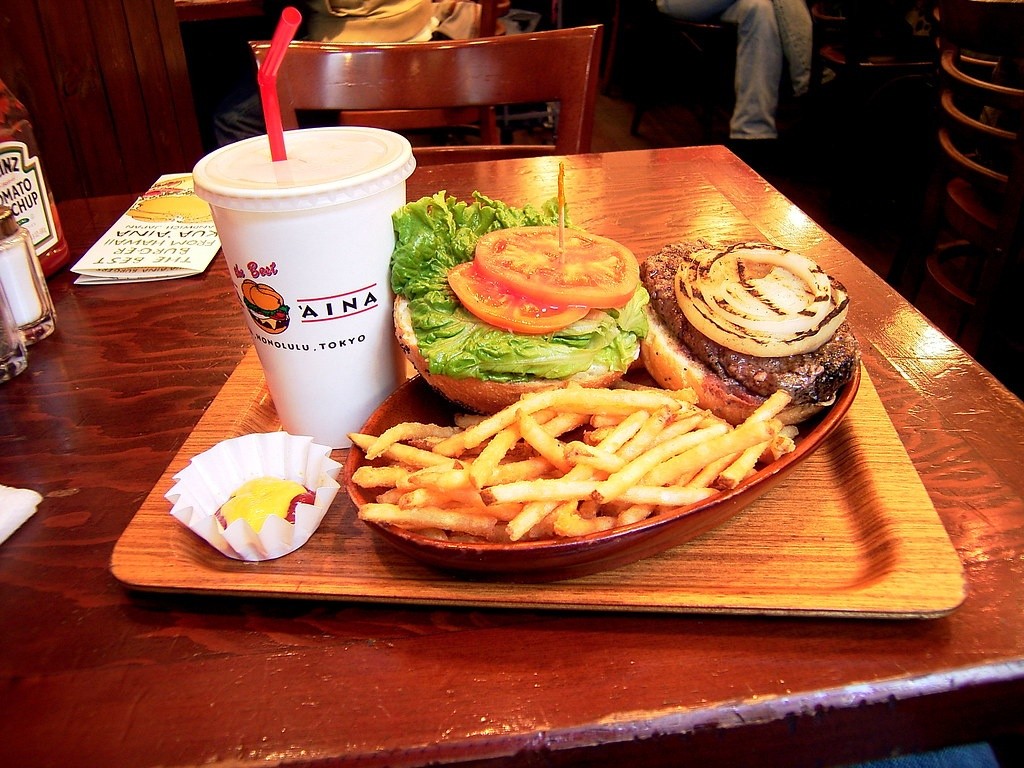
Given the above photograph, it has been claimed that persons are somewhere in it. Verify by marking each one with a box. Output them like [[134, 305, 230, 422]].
[[211, 0, 483, 147], [654, 0, 946, 178]]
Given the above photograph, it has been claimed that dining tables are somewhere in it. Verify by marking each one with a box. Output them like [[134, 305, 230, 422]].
[[0, 144, 1024, 768]]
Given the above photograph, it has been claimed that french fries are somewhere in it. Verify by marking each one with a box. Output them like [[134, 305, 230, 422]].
[[346, 383, 797, 543]]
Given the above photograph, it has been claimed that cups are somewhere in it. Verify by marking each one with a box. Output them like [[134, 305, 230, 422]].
[[191, 125, 416, 450], [0, 283, 30, 383]]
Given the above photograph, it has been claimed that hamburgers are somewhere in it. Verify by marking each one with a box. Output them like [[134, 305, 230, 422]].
[[390, 188, 862, 425]]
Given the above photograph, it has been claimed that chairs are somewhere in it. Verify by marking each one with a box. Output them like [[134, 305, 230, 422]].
[[249, 23, 606, 167]]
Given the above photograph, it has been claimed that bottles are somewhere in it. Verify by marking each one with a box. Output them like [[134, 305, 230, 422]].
[[0, 80, 70, 280], [0, 207, 58, 346]]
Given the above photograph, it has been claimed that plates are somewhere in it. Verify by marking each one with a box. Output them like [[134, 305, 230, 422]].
[[344, 358, 861, 583]]
